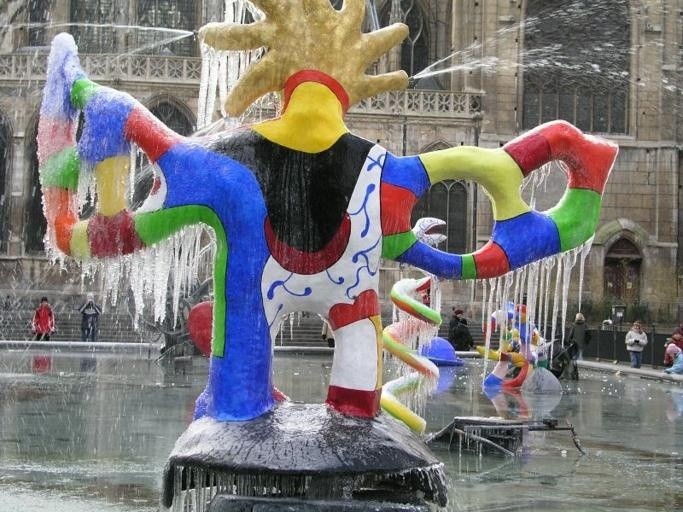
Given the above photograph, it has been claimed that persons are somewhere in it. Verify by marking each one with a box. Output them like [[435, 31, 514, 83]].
[[663, 390, 683, 422], [79, 292, 99, 340], [625, 320, 649, 368], [567, 312, 590, 360], [448, 318, 474, 351], [449, 307, 463, 333], [664, 324, 683, 374], [32, 296, 54, 341], [322, 321, 335, 346]]
